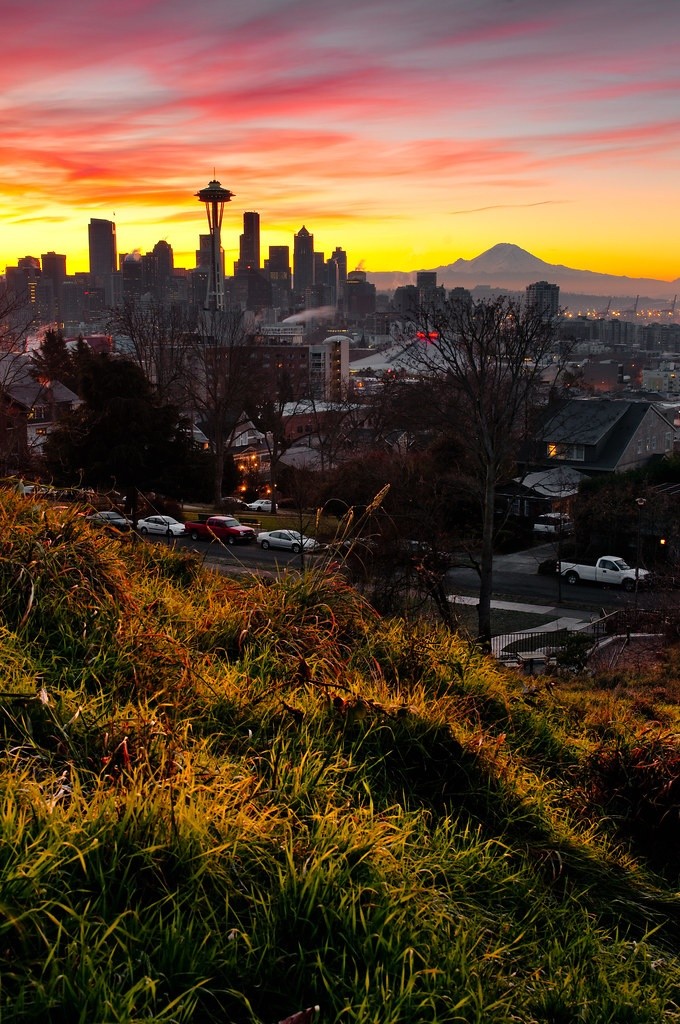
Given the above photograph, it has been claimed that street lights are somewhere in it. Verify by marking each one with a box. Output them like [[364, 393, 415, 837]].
[[631, 495, 649, 613]]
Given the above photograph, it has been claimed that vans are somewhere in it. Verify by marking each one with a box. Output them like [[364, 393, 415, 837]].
[[533, 512, 573, 540]]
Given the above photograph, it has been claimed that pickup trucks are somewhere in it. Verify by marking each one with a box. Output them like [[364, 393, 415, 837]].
[[183, 515, 256, 546], [555, 553, 650, 594]]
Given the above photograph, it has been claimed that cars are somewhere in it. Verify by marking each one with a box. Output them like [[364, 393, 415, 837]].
[[325, 533, 385, 558], [222, 496, 249, 511], [256, 529, 320, 555], [247, 500, 278, 512], [136, 516, 186, 538], [86, 511, 133, 531]]
